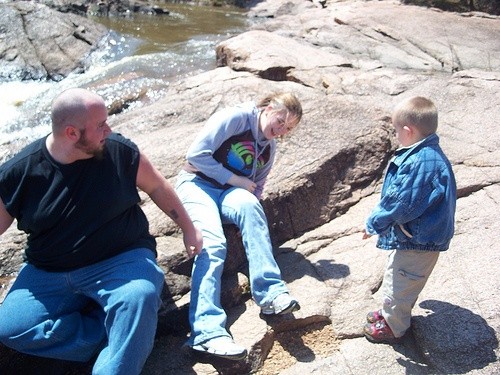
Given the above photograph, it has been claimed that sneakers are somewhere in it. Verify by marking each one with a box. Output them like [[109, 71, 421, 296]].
[[261, 292, 301, 315], [189, 335, 247, 360]]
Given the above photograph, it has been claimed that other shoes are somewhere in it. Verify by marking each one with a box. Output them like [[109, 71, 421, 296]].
[[364, 319, 402, 342], [367, 311, 384, 323]]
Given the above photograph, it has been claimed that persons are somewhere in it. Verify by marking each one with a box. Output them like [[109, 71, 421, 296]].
[[362, 97, 457, 342], [175, 93, 303, 361], [0, 88, 203, 375]]
